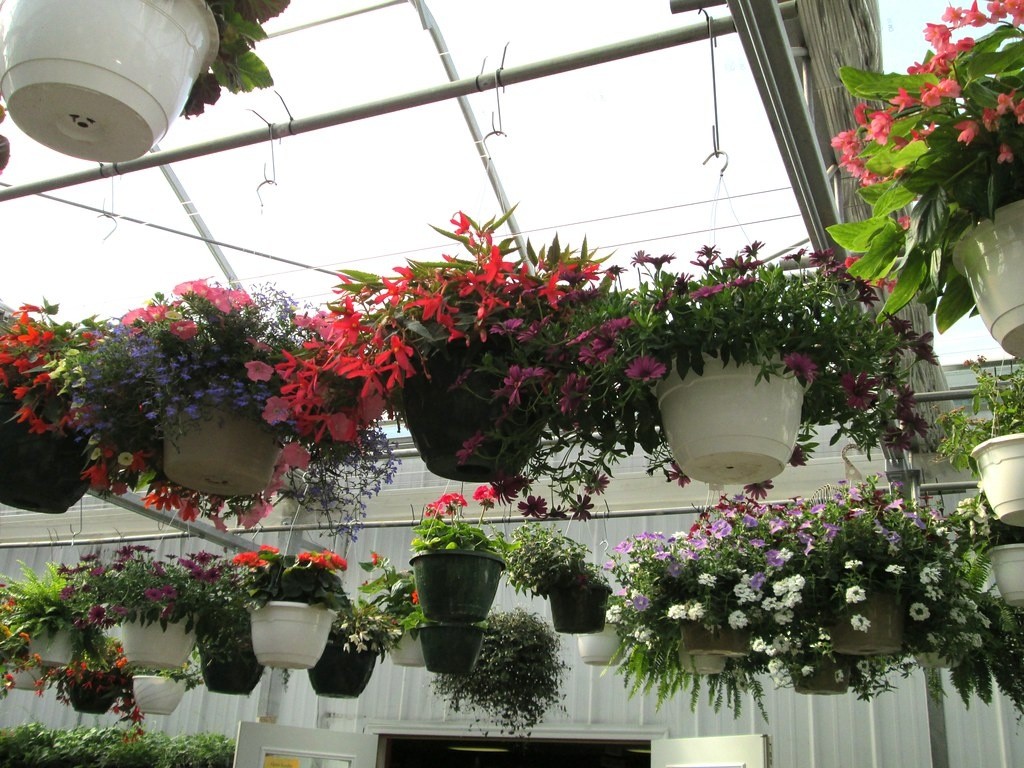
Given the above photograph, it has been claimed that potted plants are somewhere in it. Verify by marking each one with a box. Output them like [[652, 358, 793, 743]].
[[390, 626, 426, 667]]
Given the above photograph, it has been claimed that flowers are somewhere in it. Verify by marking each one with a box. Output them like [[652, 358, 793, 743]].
[[0, 0, 1024, 532], [0, 487, 599, 744], [603, 475, 1024, 724]]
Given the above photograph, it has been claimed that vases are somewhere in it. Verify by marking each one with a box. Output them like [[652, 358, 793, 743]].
[[308, 632, 378, 700], [985, 544, 1024, 607], [402, 367, 544, 483], [546, 577, 907, 696], [163, 405, 282, 496], [27, 623, 73, 664], [121, 607, 198, 666], [250, 601, 338, 668], [409, 548, 507, 622], [417, 621, 486, 674], [0, 406, 95, 515], [0, 0, 220, 164], [969, 432, 1024, 527], [952, 200, 1024, 361], [198, 648, 265, 696], [65, 670, 122, 715], [131, 674, 187, 714], [5, 658, 47, 690], [647, 348, 813, 486]]
